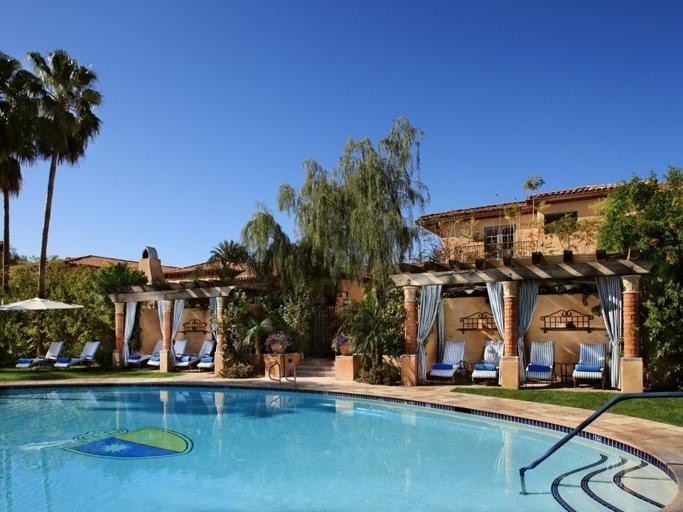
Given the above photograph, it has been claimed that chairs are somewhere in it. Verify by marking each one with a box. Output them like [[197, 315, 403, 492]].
[[15, 338, 216, 372], [429, 340, 607, 390]]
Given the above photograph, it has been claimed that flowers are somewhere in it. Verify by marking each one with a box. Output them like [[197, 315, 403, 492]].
[[330, 331, 356, 352], [264, 332, 292, 354]]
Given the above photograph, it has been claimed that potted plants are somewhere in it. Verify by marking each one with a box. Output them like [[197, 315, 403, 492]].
[[423, 200, 606, 273]]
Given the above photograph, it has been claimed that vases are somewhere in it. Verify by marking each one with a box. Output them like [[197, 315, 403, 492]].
[[270, 342, 286, 354], [340, 343, 353, 356]]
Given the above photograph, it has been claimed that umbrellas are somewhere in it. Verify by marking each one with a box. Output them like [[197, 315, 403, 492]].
[[0, 297, 84, 357]]
